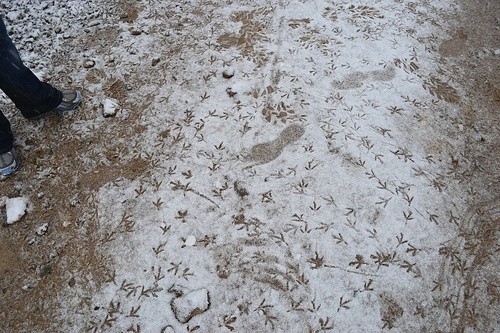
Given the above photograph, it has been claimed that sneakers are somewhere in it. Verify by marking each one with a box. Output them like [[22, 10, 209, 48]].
[[27, 88, 83, 120], [0, 146, 20, 175]]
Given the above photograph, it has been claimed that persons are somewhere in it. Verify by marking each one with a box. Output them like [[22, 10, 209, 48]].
[[0, 16, 84, 183]]
